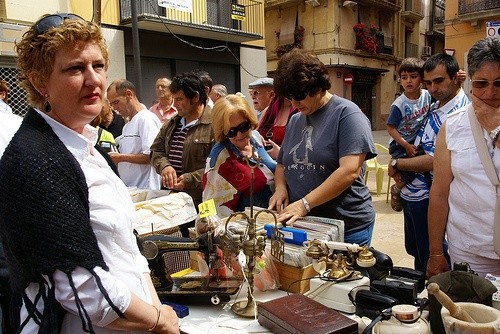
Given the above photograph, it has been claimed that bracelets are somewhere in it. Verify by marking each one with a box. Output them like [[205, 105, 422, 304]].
[[431, 255, 443, 257], [146, 305, 160, 332]]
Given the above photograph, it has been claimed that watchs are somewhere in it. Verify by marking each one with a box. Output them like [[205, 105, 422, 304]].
[[302, 198, 310, 212], [391, 159, 397, 169], [489, 126, 500, 140]]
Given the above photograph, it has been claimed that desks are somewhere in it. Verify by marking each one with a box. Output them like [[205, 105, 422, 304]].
[[176, 282, 364, 334]]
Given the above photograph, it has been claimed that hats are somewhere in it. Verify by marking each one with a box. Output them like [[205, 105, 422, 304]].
[[249, 77, 274, 89]]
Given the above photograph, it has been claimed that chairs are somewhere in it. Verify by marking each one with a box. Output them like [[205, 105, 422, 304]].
[[364, 144, 389, 198]]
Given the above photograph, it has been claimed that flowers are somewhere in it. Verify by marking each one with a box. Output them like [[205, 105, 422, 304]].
[[352, 22, 380, 55], [274, 25, 305, 57]]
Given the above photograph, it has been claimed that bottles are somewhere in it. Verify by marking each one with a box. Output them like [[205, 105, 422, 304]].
[[374, 305, 430, 334]]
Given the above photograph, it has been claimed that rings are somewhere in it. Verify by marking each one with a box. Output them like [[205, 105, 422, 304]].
[[475, 110, 476, 113]]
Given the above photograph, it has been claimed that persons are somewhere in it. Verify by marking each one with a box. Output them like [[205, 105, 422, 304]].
[[106, 80, 162, 188], [203, 95, 276, 212], [88, 98, 125, 164], [268, 51, 376, 248], [194, 69, 227, 108], [426, 36, 500, 280], [150, 78, 178, 125], [0, 13, 181, 334], [250, 78, 301, 160], [386, 53, 469, 277], [150, 72, 214, 238], [0, 81, 23, 159]]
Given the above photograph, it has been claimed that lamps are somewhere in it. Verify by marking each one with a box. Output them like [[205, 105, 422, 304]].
[[343, 1, 358, 8], [305, 0, 320, 7]]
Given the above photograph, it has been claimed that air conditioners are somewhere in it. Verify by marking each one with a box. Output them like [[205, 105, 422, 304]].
[[424, 46, 431, 55]]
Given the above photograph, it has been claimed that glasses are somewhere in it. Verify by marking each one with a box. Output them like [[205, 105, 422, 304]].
[[471, 77, 500, 88], [226, 121, 250, 138], [282, 87, 310, 102], [249, 90, 272, 98], [31, 13, 86, 42]]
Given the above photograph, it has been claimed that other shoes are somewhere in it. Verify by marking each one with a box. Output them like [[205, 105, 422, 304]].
[[391, 185, 403, 212]]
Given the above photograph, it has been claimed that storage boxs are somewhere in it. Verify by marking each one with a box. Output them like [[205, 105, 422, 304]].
[[257, 292, 359, 334], [272, 260, 325, 294]]
